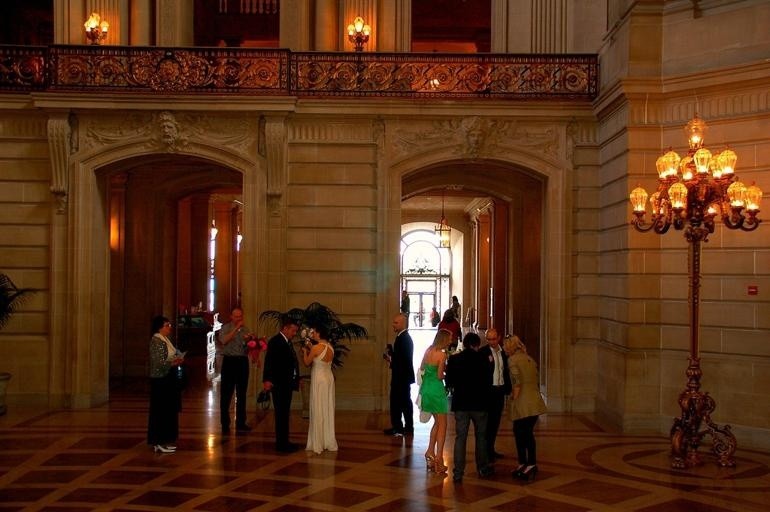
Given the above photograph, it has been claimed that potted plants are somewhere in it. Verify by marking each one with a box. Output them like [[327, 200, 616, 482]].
[[259, 302, 370, 418]]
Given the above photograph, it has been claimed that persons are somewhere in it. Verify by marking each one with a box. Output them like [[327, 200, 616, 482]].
[[219, 305, 252, 434], [384, 314, 415, 435], [301, 322, 339, 454], [503, 335, 549, 480], [431, 307, 440, 326], [450, 296, 463, 342], [438, 309, 463, 361], [478, 327, 512, 462], [147, 317, 184, 454], [262, 319, 301, 453], [417, 328, 453, 472], [444, 333, 494, 485], [400, 291, 410, 330]]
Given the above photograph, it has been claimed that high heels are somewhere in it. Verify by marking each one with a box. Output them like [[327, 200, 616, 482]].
[[512, 465, 537, 479], [153, 444, 176, 454], [425, 452, 448, 473]]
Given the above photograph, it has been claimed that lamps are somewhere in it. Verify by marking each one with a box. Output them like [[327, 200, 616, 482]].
[[347, 18, 372, 55], [85, 13, 109, 45], [434, 188, 451, 249]]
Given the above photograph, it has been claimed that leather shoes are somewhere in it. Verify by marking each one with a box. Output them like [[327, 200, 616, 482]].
[[452, 474, 461, 481], [222, 421, 250, 433], [383, 427, 414, 435], [478, 452, 504, 477]]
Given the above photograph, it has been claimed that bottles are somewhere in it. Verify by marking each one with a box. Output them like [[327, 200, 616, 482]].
[[177, 365, 183, 380]]
